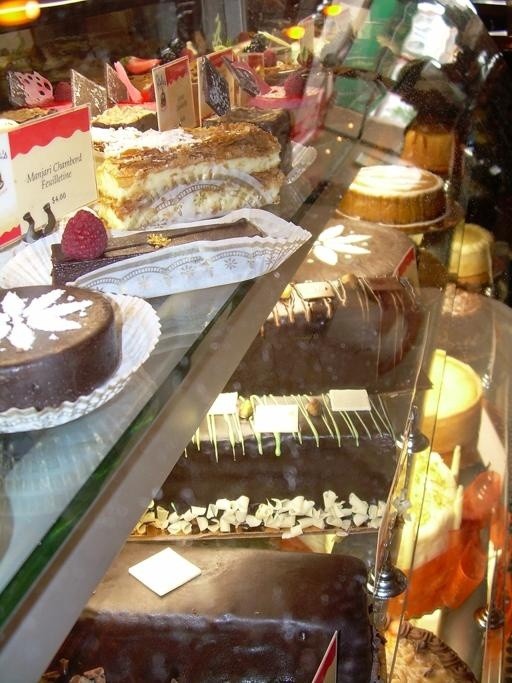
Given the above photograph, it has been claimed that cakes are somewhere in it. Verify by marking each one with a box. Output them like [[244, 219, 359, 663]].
[[128, 388, 398, 543], [336, 163, 447, 228], [416, 349, 485, 471], [448, 222, 495, 277], [401, 121, 458, 178], [387, 441, 465, 613], [377, 611, 478, 683], [417, 247, 446, 307], [220, 216, 421, 396], [432, 284, 491, 365], [36, 537, 379, 681], [0, 285, 123, 416]]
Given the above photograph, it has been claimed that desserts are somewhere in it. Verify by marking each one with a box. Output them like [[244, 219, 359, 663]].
[[1, 1, 326, 288]]
